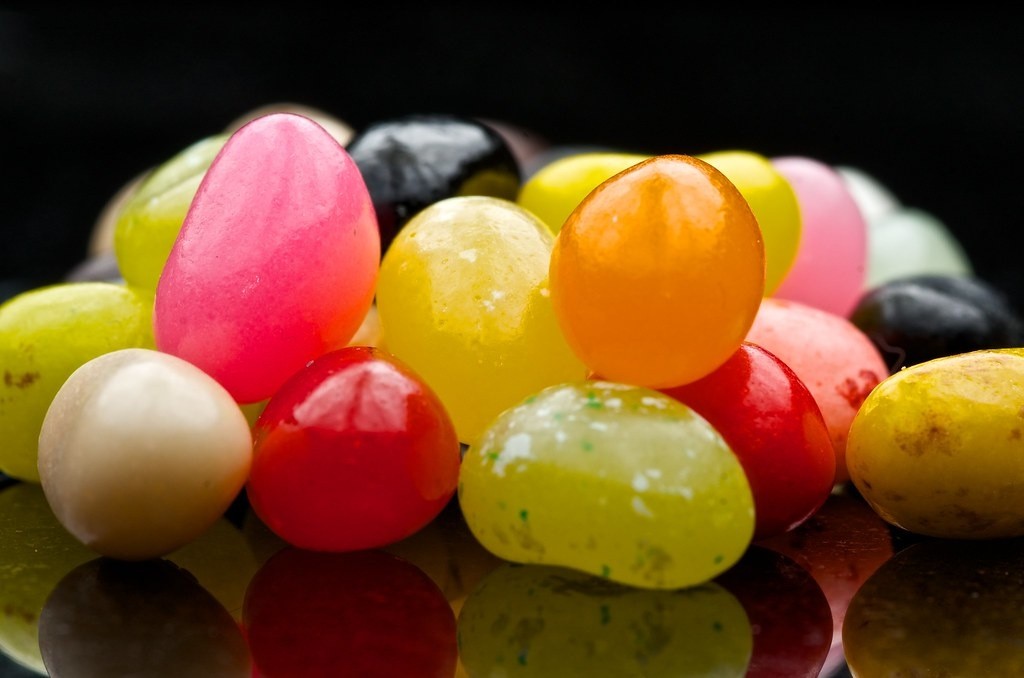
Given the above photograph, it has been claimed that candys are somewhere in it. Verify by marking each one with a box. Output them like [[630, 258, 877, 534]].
[[0, 102, 1024, 585], [0, 472, 1024, 678]]
